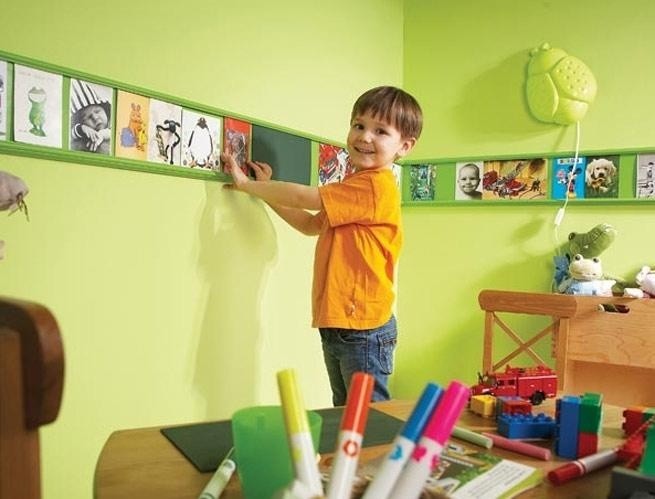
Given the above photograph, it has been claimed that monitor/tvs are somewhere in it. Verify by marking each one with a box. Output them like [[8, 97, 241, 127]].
[[352, 439, 546, 499]]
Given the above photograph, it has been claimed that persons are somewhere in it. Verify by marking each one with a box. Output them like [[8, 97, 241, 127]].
[[457, 163, 482, 201], [70, 79, 111, 152], [220, 85, 423, 407]]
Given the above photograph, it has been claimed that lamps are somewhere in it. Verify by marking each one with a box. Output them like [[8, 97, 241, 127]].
[[0, 294, 64, 498], [479, 290, 577, 391]]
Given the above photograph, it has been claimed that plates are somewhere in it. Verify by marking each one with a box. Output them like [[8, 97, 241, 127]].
[[551, 294, 655, 409]]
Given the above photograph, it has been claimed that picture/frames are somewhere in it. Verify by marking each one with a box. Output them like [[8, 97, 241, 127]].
[[94, 390, 655, 498]]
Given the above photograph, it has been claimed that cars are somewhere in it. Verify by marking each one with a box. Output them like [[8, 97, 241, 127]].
[[231, 407, 323, 498]]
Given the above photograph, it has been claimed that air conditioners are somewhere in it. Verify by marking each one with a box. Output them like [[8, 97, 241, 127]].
[[583, 157, 617, 190]]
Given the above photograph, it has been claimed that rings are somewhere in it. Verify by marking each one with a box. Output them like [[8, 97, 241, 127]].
[[199, 446, 238, 499], [278, 368, 551, 499], [547, 446, 624, 485]]
